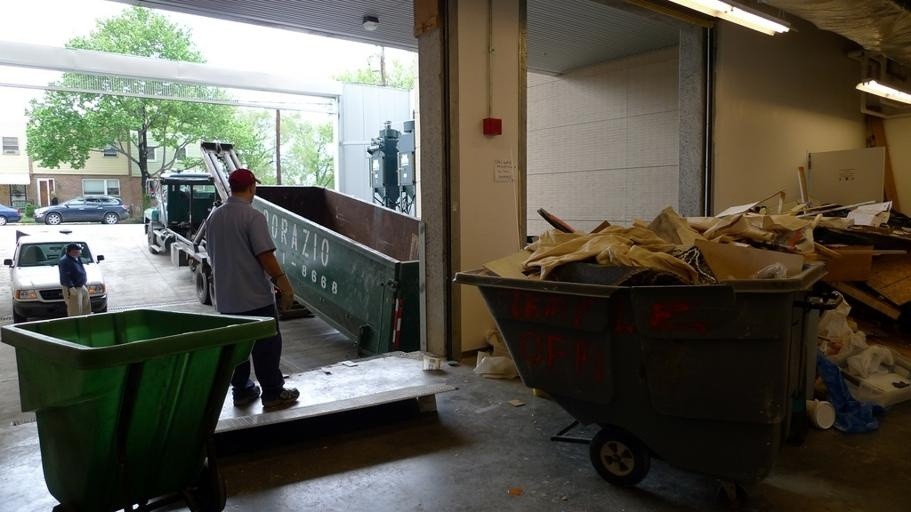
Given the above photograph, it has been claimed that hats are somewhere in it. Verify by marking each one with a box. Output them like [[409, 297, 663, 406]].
[[228, 169, 261, 186], [67, 243, 83, 250]]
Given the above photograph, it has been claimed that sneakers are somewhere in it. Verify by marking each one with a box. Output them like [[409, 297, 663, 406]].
[[261, 387, 299, 410], [233, 385, 260, 409]]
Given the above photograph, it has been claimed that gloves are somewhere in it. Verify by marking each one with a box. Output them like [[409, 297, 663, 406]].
[[270, 270, 295, 313]]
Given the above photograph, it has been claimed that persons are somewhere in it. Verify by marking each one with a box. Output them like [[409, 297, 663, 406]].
[[203, 167, 294, 411], [55, 243, 92, 317]]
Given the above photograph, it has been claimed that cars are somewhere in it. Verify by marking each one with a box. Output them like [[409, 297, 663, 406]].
[[0, 204, 20, 225], [2, 229, 111, 322]]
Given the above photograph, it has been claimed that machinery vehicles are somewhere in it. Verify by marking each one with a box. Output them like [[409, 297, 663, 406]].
[[144, 140, 316, 320]]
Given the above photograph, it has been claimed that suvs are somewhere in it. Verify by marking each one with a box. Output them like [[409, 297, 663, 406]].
[[33, 193, 129, 225]]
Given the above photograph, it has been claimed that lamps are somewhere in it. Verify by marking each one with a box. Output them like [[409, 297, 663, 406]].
[[665, 1, 792, 37], [849, 40, 910, 105]]
